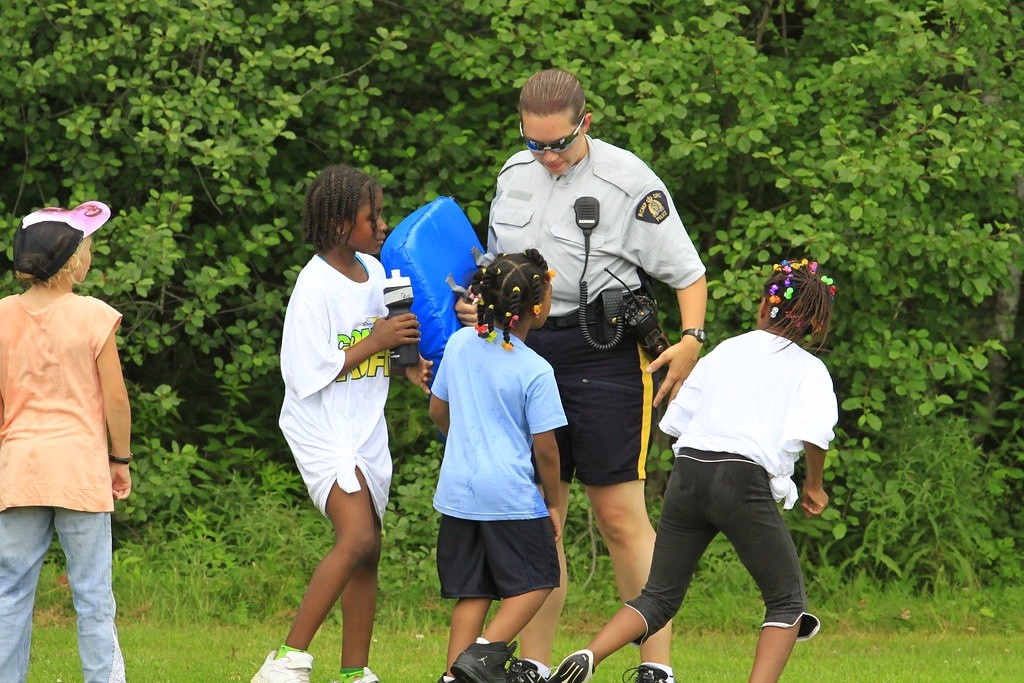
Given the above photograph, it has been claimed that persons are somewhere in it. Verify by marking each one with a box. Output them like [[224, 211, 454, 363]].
[[429, 249, 568, 683], [0, 200, 132, 683], [548, 259, 838, 683], [455, 71, 708, 683], [249, 166, 434, 683]]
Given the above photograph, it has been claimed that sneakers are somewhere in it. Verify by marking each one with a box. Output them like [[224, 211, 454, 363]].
[[441, 639, 518, 682], [622, 664, 667, 683], [353, 667, 375, 682], [507, 658, 551, 683], [250, 650, 313, 683], [546, 650, 593, 683]]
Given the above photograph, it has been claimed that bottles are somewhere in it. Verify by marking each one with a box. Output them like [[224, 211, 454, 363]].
[[383, 269, 419, 367]]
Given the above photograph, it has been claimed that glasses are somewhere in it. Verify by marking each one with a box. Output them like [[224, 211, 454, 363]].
[[519, 114, 585, 153]]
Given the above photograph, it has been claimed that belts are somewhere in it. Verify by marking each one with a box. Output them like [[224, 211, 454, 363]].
[[544, 302, 597, 328]]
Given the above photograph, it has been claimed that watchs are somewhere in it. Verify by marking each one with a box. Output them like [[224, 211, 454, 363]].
[[682, 329, 707, 343]]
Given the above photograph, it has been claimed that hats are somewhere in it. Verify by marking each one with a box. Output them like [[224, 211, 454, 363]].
[[13, 199, 110, 282]]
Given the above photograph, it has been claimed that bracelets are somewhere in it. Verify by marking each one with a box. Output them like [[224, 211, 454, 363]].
[[109, 452, 133, 464]]
